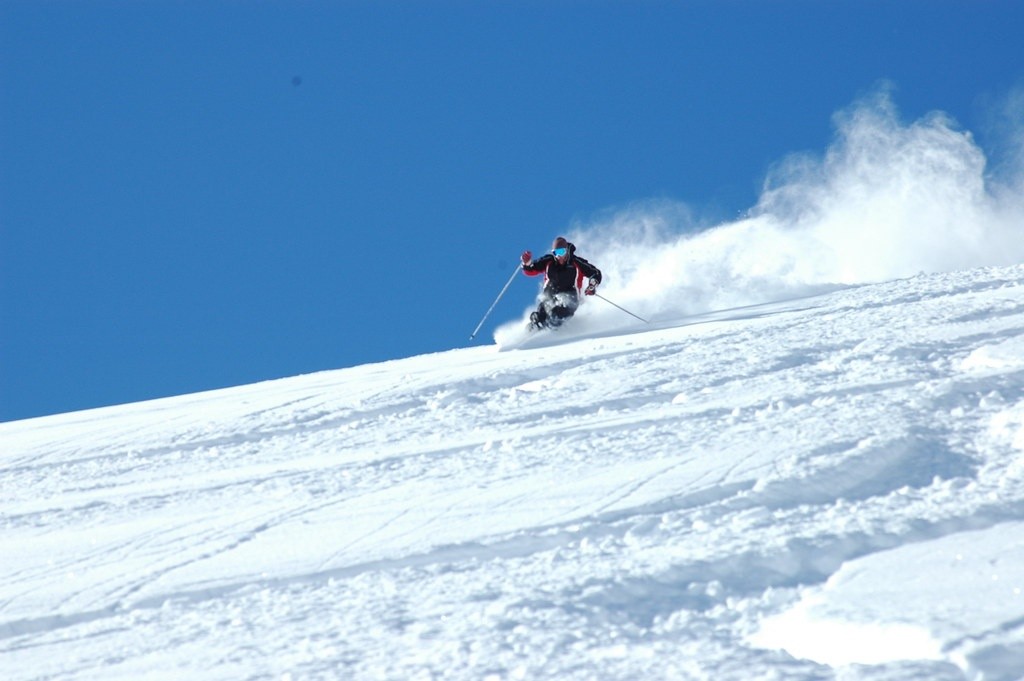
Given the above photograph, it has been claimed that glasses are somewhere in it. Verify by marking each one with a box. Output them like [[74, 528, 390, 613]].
[[551, 247, 566, 257]]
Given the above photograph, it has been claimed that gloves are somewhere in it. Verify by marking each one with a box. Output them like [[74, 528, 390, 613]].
[[584, 284, 595, 295], [520, 251, 532, 267]]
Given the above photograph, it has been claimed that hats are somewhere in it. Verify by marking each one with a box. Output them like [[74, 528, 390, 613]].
[[553, 237, 567, 248]]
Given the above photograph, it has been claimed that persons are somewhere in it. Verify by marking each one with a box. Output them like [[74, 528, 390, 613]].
[[523, 240, 602, 333]]
